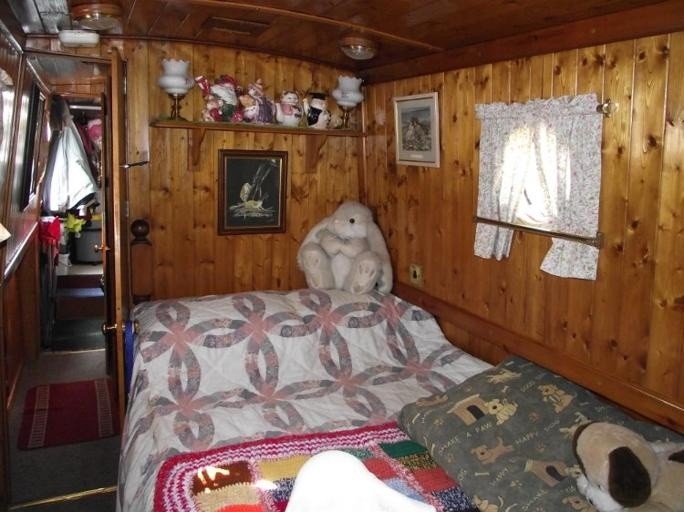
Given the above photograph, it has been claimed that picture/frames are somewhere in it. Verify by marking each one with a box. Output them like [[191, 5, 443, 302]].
[[392, 91, 441, 170], [218, 148, 289, 234], [20, 82, 47, 213]]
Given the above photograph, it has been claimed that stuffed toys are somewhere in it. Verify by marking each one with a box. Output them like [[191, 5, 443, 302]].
[[295, 199, 395, 296], [569, 418, 684, 512], [302, 92, 331, 131], [193, 73, 244, 122], [274, 88, 304, 128], [237, 77, 278, 125]]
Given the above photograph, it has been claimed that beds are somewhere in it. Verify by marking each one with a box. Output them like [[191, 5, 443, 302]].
[[116, 220, 684, 512]]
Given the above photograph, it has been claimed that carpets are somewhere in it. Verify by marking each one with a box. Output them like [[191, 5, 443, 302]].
[[16, 378, 120, 450]]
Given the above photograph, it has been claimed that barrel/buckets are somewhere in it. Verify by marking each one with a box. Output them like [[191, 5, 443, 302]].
[[55, 252, 72, 276]]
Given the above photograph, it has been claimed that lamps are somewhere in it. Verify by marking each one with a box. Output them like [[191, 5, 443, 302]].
[[157, 58, 195, 120], [70, 4, 123, 31], [337, 30, 379, 61], [333, 76, 365, 128]]
